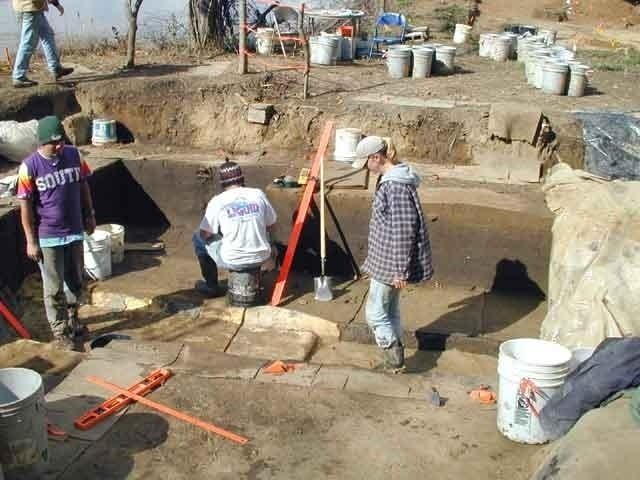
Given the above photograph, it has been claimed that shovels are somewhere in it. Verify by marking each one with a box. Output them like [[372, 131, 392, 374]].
[[313, 156, 336, 301]]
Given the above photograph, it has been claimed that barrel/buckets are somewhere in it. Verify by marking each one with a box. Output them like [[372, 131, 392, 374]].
[[227, 266, 262, 308], [92, 119, 117, 147], [94, 224, 125, 264], [1, 368, 50, 469], [334, 126, 361, 162], [498, 337, 599, 449], [83, 230, 112, 281]]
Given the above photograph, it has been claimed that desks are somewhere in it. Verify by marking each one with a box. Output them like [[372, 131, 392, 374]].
[[304, 9, 368, 40]]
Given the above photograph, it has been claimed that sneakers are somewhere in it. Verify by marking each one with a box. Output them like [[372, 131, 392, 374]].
[[54, 67, 74, 79], [195, 279, 218, 295], [519, 376, 551, 416], [57, 316, 90, 352], [12, 76, 37, 88]]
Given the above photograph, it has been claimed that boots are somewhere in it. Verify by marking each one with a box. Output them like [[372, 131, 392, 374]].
[[371, 346, 406, 373]]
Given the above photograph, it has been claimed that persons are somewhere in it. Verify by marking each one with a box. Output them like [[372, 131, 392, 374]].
[[351, 135, 434, 374], [17, 117, 96, 350], [193, 162, 280, 297], [12, 0, 74, 87]]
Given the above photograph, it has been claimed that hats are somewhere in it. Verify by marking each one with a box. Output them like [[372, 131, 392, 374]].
[[351, 136, 388, 169], [219, 162, 245, 187], [37, 115, 65, 145]]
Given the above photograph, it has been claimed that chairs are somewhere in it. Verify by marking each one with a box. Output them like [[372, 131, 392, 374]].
[[271, 6, 299, 57], [369, 13, 407, 64]]
